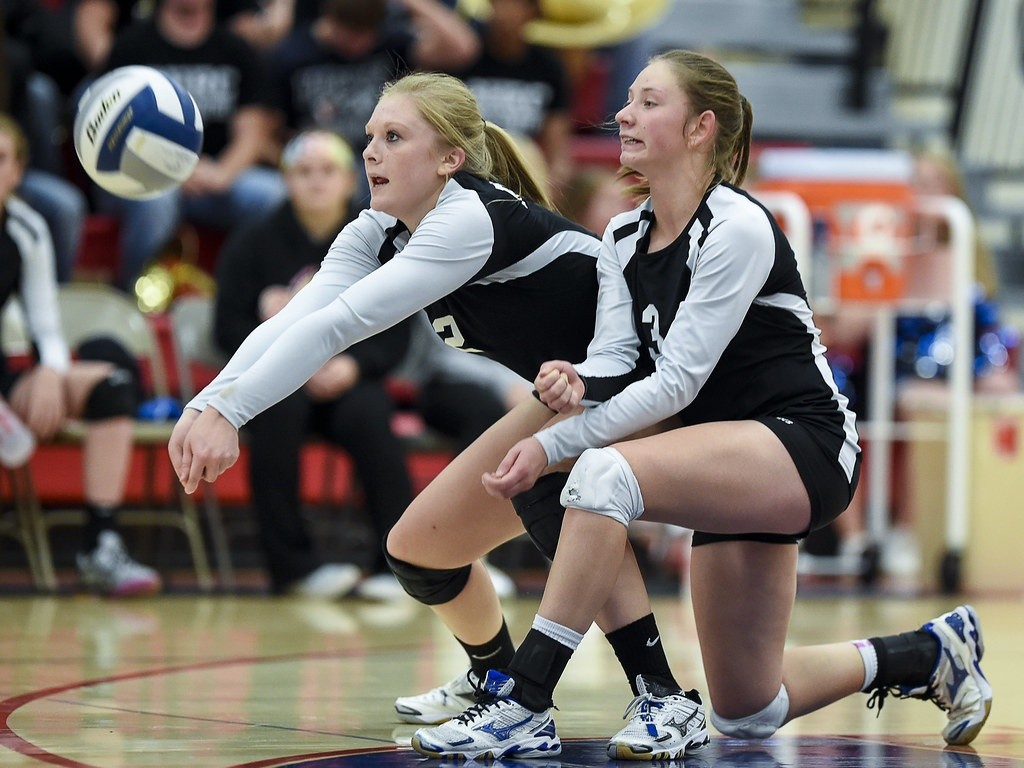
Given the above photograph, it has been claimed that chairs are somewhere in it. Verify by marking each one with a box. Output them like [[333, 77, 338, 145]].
[[169, 296, 340, 599], [8, 281, 208, 591]]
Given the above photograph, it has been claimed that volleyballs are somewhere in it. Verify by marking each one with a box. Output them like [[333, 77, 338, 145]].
[[72, 63, 205, 201]]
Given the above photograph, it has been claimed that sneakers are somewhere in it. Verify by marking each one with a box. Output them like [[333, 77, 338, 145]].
[[411, 666, 562, 758], [606, 674, 712, 759], [395, 665, 486, 725], [868, 604, 993, 745]]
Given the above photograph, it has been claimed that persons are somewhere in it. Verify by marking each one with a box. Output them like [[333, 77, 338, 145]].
[[0, 0, 1007, 598], [168, 70, 710, 759], [410, 51, 993, 757]]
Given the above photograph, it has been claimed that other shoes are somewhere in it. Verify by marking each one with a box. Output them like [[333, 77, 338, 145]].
[[287, 560, 362, 600], [74, 529, 164, 597], [355, 572, 405, 600]]
